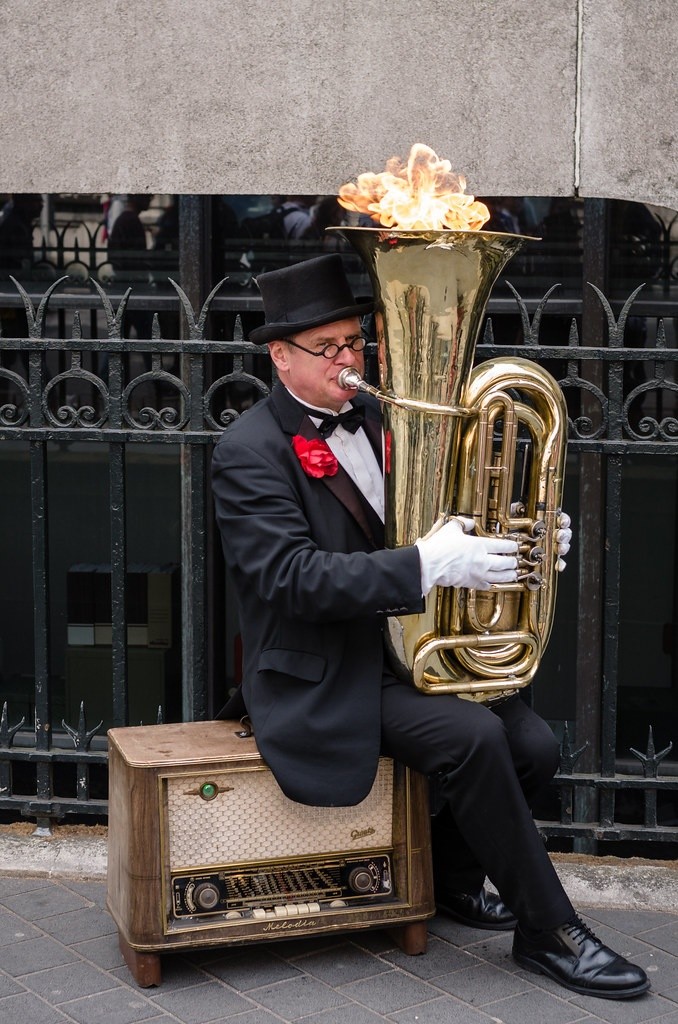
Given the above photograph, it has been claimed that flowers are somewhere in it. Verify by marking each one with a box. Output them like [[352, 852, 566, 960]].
[[292, 436, 338, 478]]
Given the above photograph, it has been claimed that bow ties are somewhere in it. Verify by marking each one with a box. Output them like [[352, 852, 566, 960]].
[[299, 400, 366, 440]]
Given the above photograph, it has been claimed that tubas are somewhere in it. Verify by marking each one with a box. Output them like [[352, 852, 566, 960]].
[[323, 227, 569, 705]]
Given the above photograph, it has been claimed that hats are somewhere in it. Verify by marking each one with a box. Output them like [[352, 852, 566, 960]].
[[248, 253, 379, 345]]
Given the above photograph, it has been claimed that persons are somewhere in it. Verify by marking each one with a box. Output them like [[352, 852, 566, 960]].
[[477, 196, 537, 242], [214, 253, 650, 1001], [2, 195, 361, 295]]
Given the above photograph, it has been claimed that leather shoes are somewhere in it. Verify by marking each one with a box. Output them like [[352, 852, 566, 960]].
[[435, 886, 518, 930], [512, 913, 651, 998]]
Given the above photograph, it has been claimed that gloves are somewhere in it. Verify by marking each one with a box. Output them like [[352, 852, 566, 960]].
[[414, 516, 518, 599], [413, 501, 572, 572]]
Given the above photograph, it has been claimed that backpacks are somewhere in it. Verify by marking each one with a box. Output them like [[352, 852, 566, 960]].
[[238, 206, 308, 266]]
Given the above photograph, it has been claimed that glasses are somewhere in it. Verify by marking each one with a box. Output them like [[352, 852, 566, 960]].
[[286, 337, 367, 359]]
